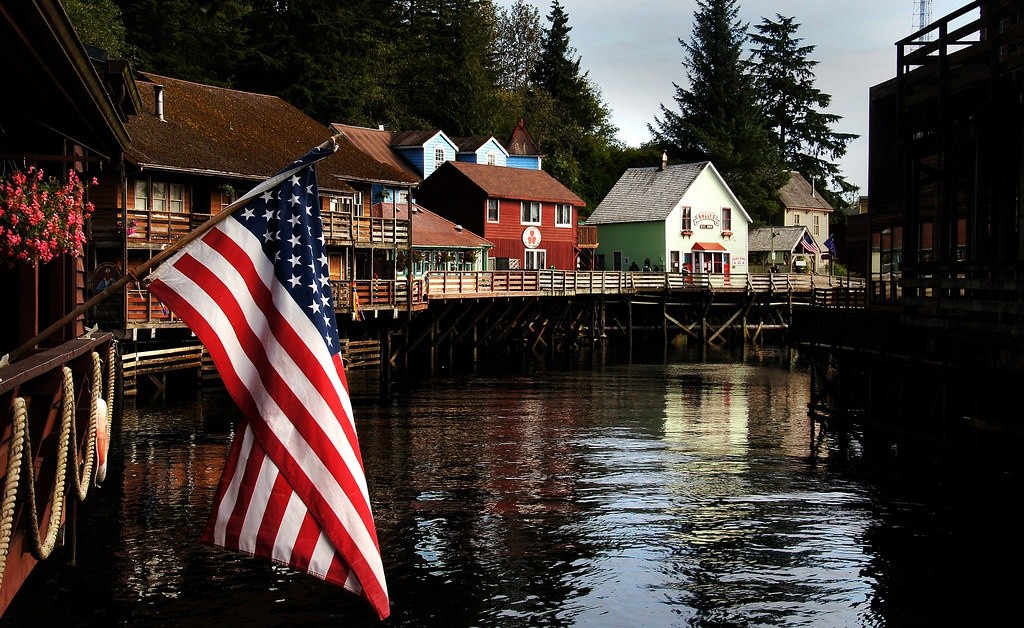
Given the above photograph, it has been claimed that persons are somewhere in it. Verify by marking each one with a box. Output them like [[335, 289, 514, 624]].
[[653, 264, 660, 284], [682, 263, 689, 285], [629, 262, 639, 271], [643, 265, 651, 284]]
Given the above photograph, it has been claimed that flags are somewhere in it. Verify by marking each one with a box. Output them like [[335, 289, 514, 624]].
[[145, 145, 391, 620], [800, 235, 817, 253], [824, 236, 836, 257]]
